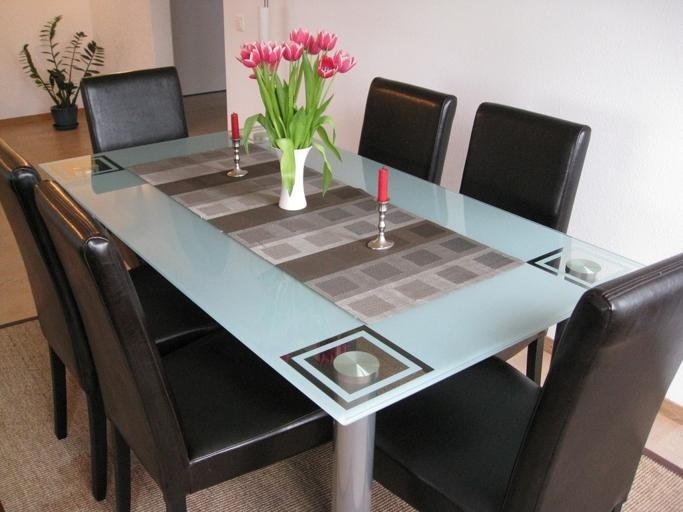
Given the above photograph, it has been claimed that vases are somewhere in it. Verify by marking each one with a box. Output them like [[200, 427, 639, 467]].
[[271, 144, 314, 212]]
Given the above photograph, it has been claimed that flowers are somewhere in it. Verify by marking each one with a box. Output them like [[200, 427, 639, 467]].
[[234, 29, 357, 196]]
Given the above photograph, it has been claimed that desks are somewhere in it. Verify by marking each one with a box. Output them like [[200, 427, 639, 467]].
[[37, 124, 648, 512]]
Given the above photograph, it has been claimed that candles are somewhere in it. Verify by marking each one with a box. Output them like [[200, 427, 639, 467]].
[[231, 113, 239, 139], [379, 168, 388, 202]]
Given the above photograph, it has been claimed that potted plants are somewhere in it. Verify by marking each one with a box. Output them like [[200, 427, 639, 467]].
[[18, 15, 105, 130]]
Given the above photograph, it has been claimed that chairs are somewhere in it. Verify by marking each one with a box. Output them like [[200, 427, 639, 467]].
[[79, 65, 189, 154], [456, 101, 591, 385], [356, 77, 458, 187], [32, 177, 335, 512], [372, 250, 683, 512], [0, 164, 223, 503]]
